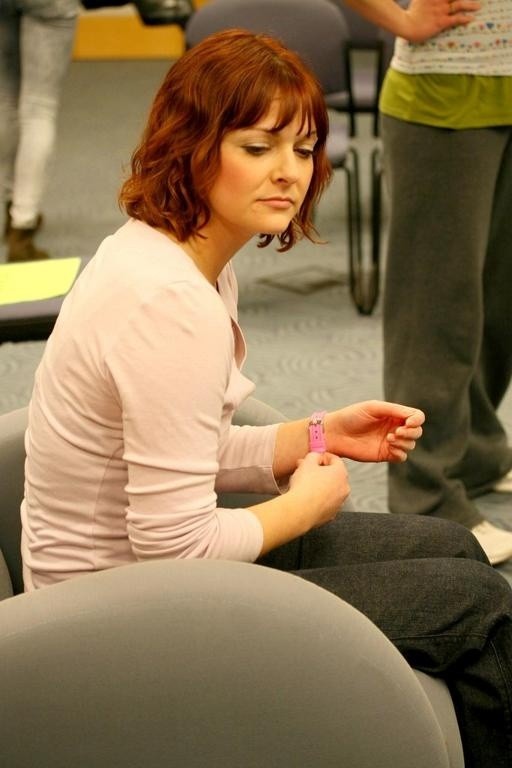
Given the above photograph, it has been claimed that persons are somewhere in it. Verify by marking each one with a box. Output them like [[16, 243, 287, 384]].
[[342, 0, 512, 566], [0, 0, 79, 261], [19, 29, 512, 767]]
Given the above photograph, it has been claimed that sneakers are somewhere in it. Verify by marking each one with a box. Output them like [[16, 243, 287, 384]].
[[470, 520, 512, 566]]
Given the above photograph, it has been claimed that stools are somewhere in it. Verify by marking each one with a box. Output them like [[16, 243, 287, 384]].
[[3, 250, 89, 346]]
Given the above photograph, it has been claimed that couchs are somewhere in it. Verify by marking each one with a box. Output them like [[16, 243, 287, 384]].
[[1, 384, 471, 768]]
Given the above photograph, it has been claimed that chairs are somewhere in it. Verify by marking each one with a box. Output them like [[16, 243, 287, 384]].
[[186, 2, 365, 318]]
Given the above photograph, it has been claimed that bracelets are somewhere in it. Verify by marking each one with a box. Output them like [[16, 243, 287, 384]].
[[309, 412, 326, 453]]
